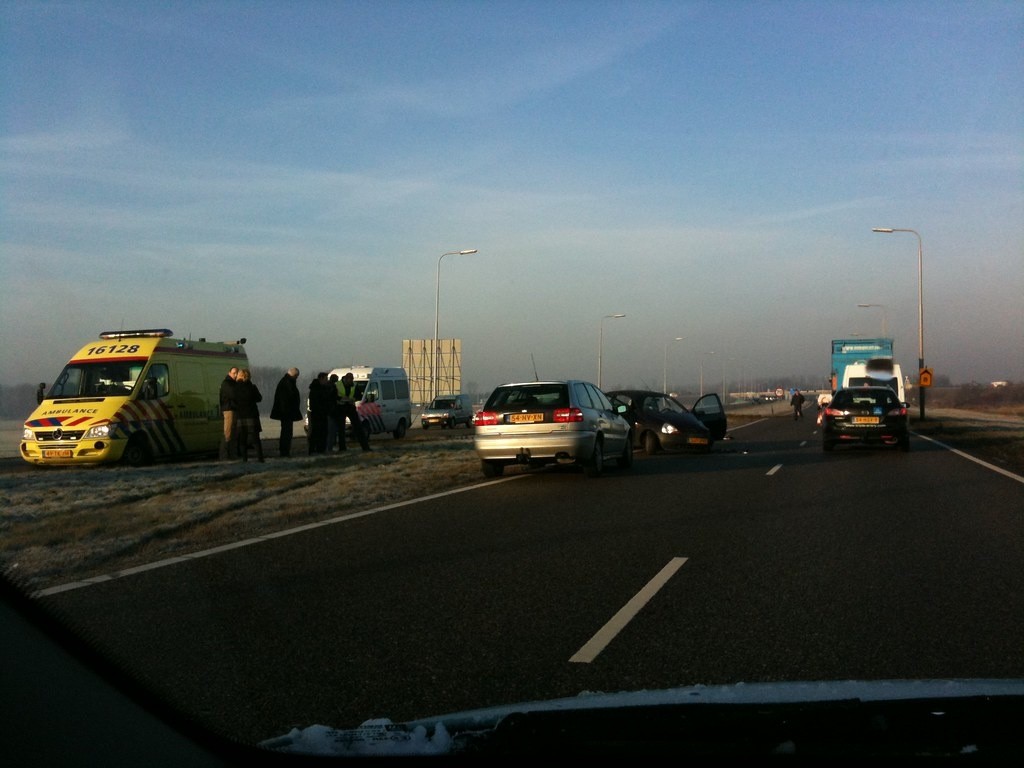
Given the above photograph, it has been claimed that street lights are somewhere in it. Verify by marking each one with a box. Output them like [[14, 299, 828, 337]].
[[872, 227, 925, 420], [700, 352, 716, 407], [723, 357, 735, 407], [433, 250, 478, 408], [858, 303, 886, 340], [597, 314, 626, 388], [664, 338, 684, 407]]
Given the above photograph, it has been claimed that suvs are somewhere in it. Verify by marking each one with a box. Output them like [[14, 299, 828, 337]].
[[818, 393, 833, 408]]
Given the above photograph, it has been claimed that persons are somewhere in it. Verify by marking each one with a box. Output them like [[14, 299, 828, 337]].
[[219, 368, 264, 462], [308, 373, 375, 456], [790, 389, 805, 420], [270, 368, 303, 457]]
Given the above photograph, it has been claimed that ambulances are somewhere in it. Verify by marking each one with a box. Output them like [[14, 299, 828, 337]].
[[17, 328, 251, 470]]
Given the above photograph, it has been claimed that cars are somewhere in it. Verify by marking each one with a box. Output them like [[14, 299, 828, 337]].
[[751, 395, 775, 404], [603, 391, 728, 457], [821, 386, 911, 451], [473, 380, 634, 478]]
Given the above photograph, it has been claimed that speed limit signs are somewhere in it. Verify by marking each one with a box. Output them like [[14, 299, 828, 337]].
[[775, 388, 784, 398]]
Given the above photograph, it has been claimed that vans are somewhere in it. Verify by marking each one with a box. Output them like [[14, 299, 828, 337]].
[[843, 363, 906, 409], [303, 364, 412, 440], [420, 394, 473, 430]]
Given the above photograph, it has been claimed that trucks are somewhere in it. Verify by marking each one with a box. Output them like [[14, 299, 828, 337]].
[[829, 339, 892, 399]]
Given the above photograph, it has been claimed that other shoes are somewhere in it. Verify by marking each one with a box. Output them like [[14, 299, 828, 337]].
[[339, 446, 348, 451], [362, 448, 374, 454]]
